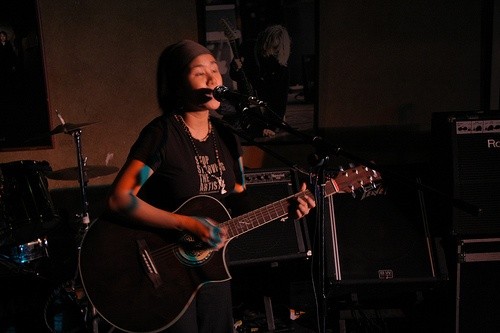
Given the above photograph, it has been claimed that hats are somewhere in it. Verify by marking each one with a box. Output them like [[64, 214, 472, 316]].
[[155, 39, 213, 113]]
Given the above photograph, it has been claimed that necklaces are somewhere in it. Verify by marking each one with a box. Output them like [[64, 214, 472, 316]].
[[182, 115, 227, 197]]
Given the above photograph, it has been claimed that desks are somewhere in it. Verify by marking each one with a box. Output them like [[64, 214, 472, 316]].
[[454, 234, 500, 333]]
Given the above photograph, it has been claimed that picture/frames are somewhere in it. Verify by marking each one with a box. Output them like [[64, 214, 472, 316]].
[[196, 0, 319, 145], [0, 0, 55, 151]]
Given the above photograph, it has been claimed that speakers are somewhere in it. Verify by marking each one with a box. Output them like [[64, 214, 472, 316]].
[[431, 110, 500, 240], [224, 168, 310, 266], [450, 237, 500, 333], [322, 166, 450, 333]]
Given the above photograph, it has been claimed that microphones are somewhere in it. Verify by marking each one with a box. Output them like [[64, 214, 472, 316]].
[[214, 85, 269, 108]]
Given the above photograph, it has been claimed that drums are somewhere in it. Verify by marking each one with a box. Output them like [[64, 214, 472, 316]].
[[79, 162, 384, 333], [0, 159, 60, 262]]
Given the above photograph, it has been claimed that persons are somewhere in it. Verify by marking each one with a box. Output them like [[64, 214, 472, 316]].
[[0, 30, 14, 58], [111, 39, 317, 333]]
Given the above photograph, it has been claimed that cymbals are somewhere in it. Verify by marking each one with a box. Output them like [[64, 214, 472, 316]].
[[40, 120, 98, 139], [46, 164, 119, 181]]
